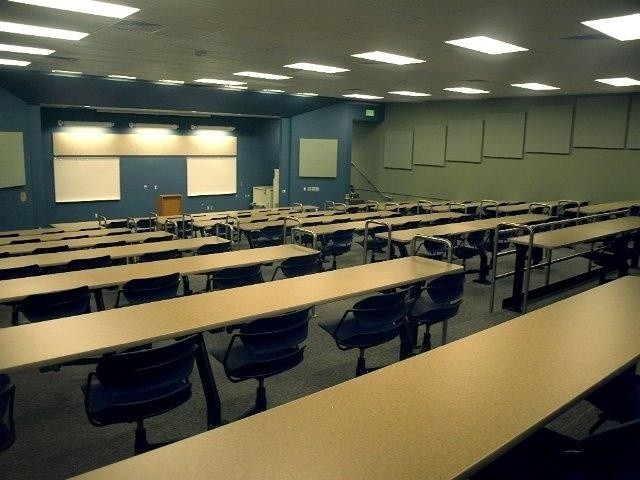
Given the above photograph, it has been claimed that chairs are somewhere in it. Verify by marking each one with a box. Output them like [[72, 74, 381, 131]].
[[1, 199, 639, 480]]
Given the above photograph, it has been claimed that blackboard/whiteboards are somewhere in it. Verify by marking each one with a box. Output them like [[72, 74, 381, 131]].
[[186, 156, 237, 197], [53, 157, 121, 203]]
